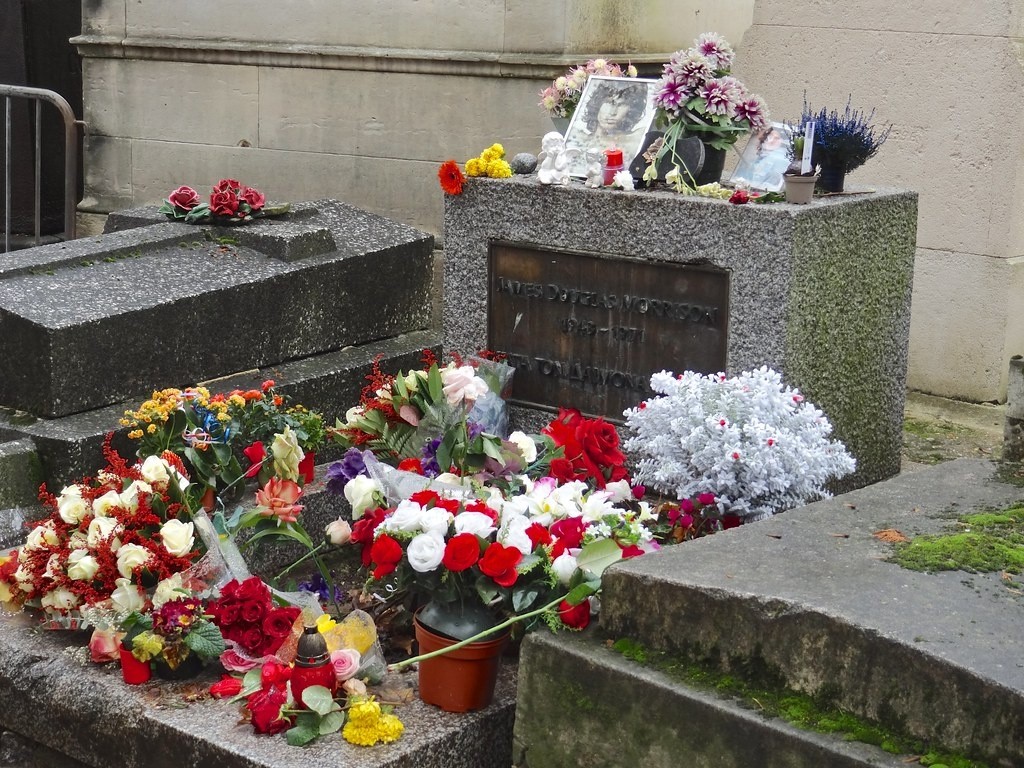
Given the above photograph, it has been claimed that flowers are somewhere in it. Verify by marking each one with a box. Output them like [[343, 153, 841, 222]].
[[162, 181, 262, 224], [0, 347, 856, 748], [438, 33, 892, 196]]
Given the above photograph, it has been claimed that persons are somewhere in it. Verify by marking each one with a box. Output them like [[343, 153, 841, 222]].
[[572, 79, 648, 166], [734, 126, 791, 190]]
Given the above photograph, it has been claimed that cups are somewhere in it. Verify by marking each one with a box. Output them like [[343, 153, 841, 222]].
[[113, 637, 150, 684]]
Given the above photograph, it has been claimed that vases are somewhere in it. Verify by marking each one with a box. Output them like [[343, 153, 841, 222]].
[[692, 147, 730, 187], [413, 605, 512, 713], [782, 174, 817, 205]]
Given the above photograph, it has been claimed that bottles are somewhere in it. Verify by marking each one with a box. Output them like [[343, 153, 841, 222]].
[[291, 624, 337, 707]]
